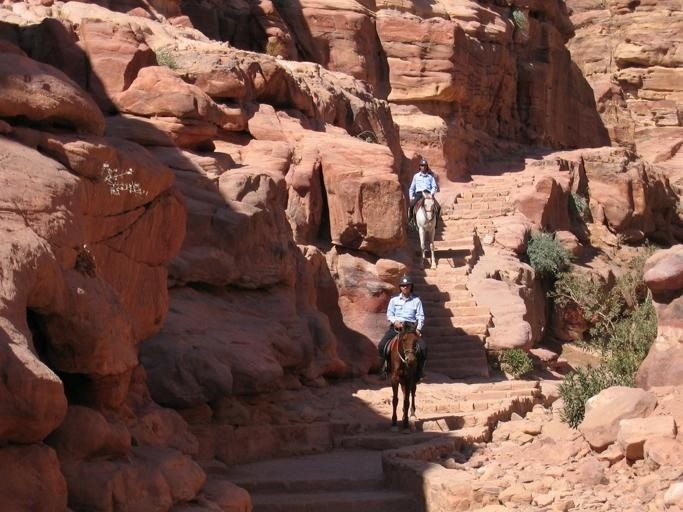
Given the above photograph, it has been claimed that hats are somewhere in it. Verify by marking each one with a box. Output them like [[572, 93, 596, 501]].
[[419, 160, 428, 165], [399, 275, 413, 286]]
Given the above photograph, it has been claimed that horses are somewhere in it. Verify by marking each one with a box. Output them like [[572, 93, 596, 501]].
[[385, 318, 421, 433], [415, 191, 437, 269]]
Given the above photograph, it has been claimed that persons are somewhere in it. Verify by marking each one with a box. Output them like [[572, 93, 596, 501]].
[[378, 275, 429, 378], [407, 160, 442, 230]]
